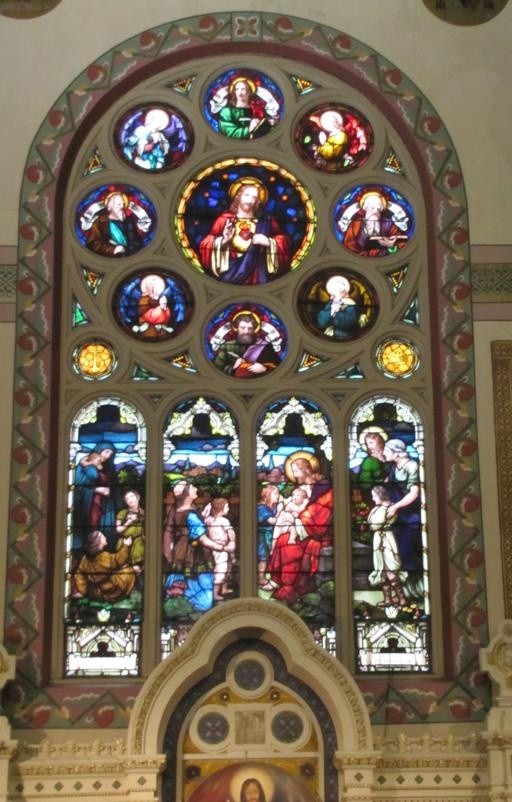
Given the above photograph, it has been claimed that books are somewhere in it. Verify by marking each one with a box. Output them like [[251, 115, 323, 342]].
[[365, 235, 408, 248]]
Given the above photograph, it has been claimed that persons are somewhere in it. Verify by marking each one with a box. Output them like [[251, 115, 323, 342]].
[[344, 192, 408, 257], [198, 175, 291, 285], [217, 76, 269, 139], [306, 113, 352, 169], [315, 275, 361, 337], [84, 190, 145, 257], [124, 117, 170, 171], [126, 276, 174, 339], [213, 311, 282, 379]]
